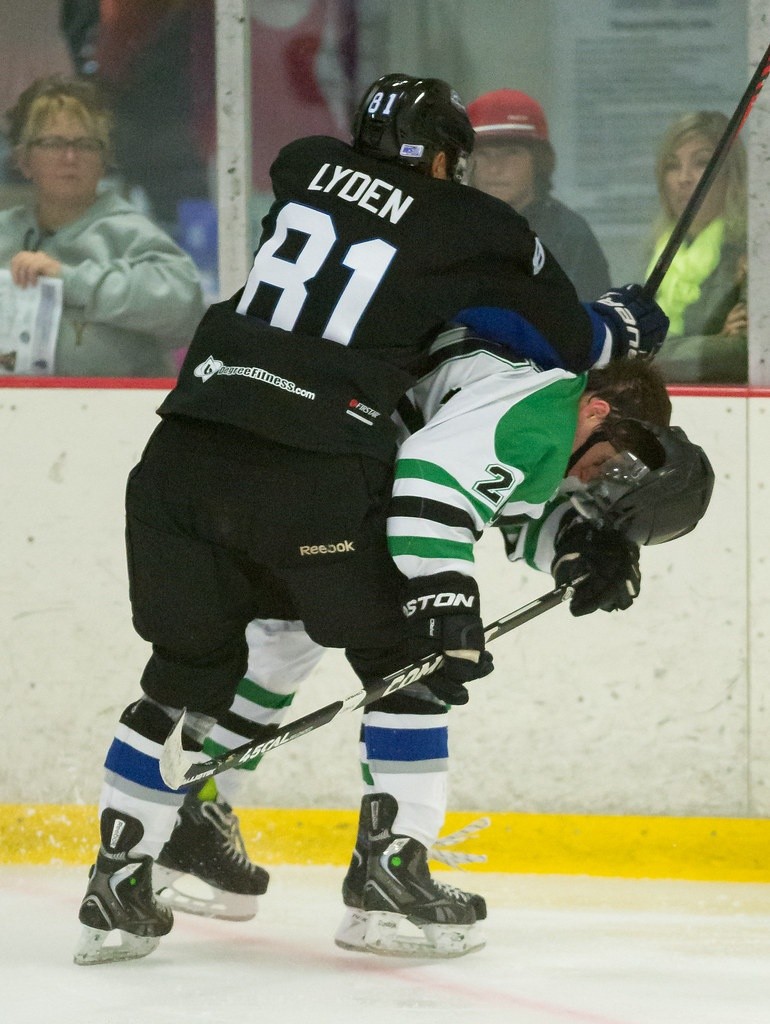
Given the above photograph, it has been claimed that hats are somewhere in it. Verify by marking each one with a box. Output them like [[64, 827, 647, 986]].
[[458, 91, 548, 142]]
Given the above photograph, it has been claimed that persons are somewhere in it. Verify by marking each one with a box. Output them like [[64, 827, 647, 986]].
[[75, 73, 715, 964], [0, 74, 745, 378]]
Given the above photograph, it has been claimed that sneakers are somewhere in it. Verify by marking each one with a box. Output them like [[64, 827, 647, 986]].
[[334, 792, 489, 958], [89, 792, 269, 921], [73, 807, 173, 962]]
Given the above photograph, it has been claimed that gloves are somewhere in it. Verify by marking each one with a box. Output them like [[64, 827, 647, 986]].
[[398, 571, 494, 705], [550, 507, 641, 616], [590, 282, 668, 364]]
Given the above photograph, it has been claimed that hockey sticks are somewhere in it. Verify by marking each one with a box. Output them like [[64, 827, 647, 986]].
[[160, 570, 590, 794], [643, 43, 770, 300]]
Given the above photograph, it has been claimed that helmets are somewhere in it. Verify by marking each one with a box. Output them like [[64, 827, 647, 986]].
[[348, 74, 475, 180], [596, 426, 714, 545]]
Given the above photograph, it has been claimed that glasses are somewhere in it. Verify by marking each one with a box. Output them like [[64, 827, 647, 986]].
[[27, 135, 106, 154]]
